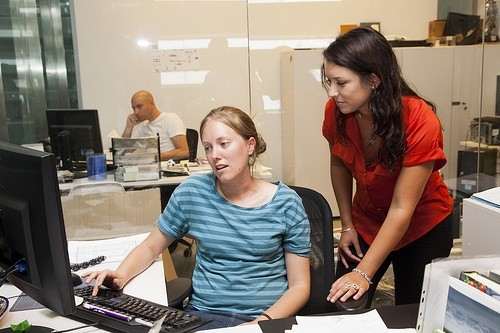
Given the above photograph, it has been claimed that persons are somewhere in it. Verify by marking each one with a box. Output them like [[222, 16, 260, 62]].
[[81, 106, 311, 333], [320, 26, 454, 306], [121, 90, 189, 162]]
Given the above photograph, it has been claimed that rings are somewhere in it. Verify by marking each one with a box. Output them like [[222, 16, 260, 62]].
[[353, 284, 358, 290], [345, 283, 351, 290]]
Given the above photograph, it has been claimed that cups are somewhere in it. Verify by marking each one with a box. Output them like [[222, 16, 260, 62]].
[[86, 153, 107, 181]]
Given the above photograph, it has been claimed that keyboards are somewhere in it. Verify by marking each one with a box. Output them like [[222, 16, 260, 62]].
[[69, 285, 214, 333], [72, 161, 114, 171]]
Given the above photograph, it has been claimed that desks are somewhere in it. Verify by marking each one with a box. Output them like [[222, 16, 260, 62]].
[[0, 230, 419, 333], [61, 151, 278, 191]]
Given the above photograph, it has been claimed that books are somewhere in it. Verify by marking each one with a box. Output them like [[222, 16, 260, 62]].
[[459, 271, 500, 296]]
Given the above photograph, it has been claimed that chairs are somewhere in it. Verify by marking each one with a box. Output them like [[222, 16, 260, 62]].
[[170, 127, 201, 256], [282, 179, 367, 315], [64, 181, 127, 195]]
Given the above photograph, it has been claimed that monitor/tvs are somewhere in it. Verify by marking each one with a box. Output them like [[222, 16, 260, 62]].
[[46, 108, 103, 173], [0, 141, 77, 315]]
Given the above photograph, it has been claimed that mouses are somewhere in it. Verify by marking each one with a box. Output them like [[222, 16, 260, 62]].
[[71, 273, 82, 287]]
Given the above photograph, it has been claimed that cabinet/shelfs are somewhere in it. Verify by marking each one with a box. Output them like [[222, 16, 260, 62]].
[[274, 42, 500, 223]]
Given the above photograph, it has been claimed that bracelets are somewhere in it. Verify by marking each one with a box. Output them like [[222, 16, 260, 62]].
[[262, 313, 271, 321], [340, 227, 353, 233], [352, 268, 373, 284]]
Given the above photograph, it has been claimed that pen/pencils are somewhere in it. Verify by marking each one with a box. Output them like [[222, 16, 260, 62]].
[[83, 304, 130, 321]]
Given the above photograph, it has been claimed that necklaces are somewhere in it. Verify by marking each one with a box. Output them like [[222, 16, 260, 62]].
[[357, 111, 368, 120]]
